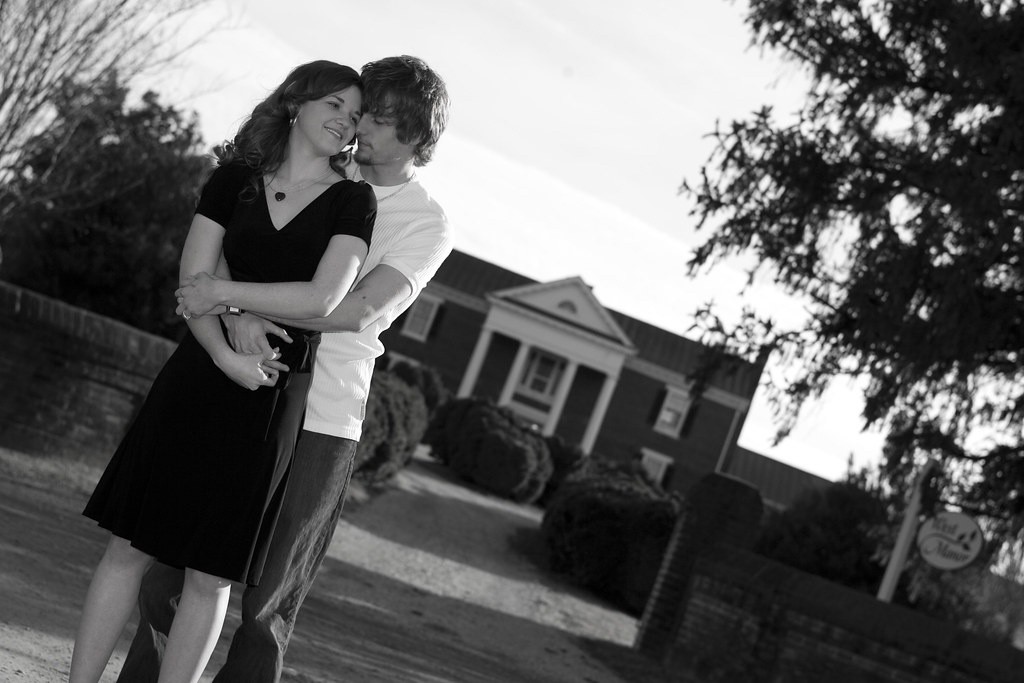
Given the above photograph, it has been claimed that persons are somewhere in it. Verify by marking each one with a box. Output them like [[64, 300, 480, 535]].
[[64, 61, 377, 683], [115, 54, 458, 683]]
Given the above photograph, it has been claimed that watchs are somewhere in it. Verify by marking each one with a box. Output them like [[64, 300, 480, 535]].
[[225, 306, 246, 316]]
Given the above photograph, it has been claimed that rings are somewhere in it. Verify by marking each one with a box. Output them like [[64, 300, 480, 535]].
[[183, 310, 191, 320]]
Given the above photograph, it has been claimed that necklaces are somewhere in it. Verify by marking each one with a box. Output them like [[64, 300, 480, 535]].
[[351, 160, 415, 204], [264, 165, 335, 202]]
